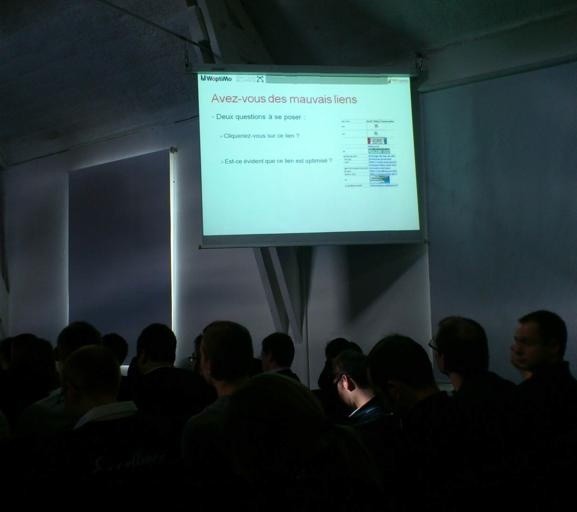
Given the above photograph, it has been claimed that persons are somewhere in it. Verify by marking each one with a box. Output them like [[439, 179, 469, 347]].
[[1, 310, 576, 511]]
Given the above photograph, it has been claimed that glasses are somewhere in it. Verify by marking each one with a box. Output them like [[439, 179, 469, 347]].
[[428, 338, 441, 353], [332, 374, 344, 387], [188, 356, 199, 363]]
